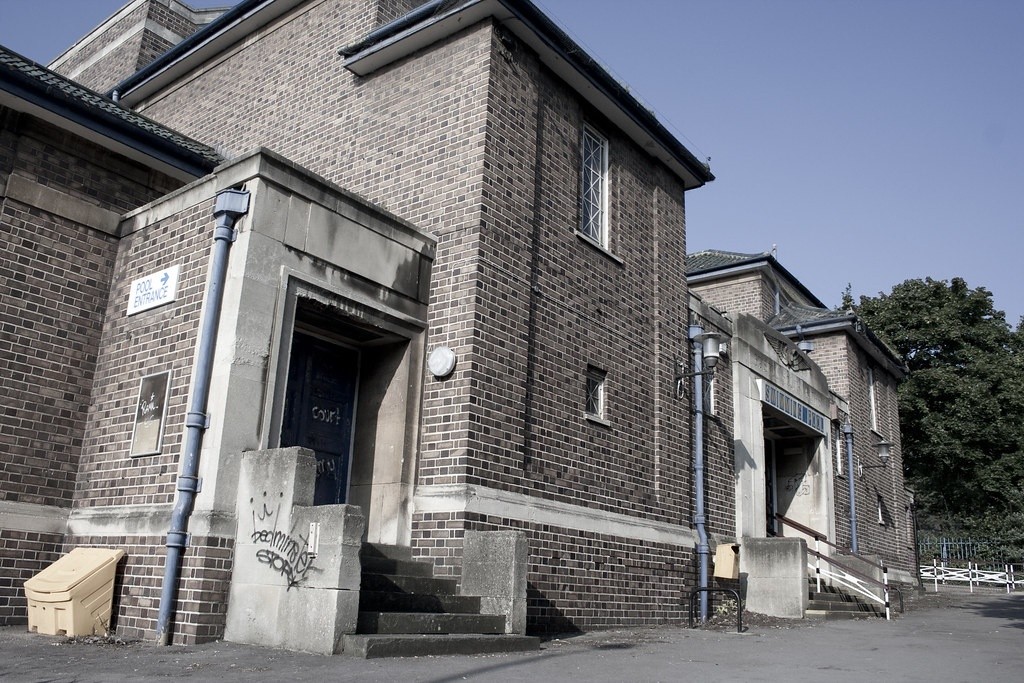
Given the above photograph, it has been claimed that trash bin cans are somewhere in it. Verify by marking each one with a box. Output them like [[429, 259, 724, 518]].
[[22, 540, 127, 640]]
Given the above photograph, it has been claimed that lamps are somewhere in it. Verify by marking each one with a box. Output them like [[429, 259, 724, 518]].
[[672, 331, 729, 397], [858, 440, 898, 478], [427, 347, 455, 376]]
